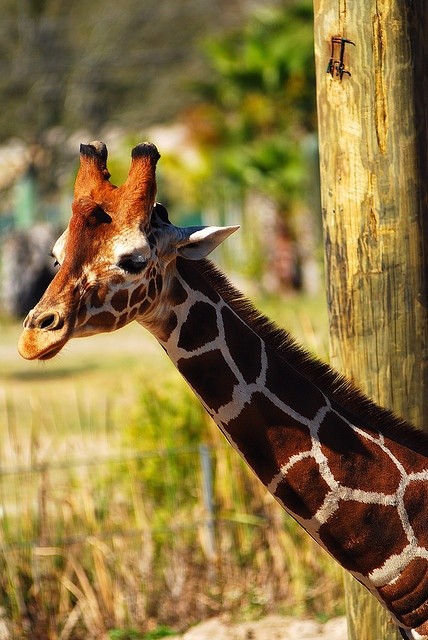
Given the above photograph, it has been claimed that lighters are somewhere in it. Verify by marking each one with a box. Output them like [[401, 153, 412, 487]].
[[16, 139, 426, 639]]
